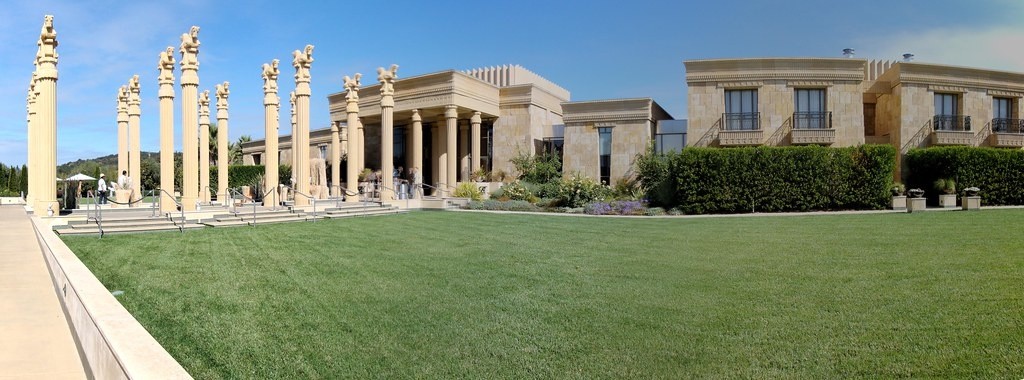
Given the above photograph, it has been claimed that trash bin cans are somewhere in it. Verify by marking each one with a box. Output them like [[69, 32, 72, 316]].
[[399, 179, 409, 199]]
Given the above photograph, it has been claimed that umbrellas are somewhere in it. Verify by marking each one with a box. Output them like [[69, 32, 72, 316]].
[[57, 173, 99, 198]]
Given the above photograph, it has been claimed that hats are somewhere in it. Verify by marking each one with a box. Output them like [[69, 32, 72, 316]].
[[100, 173, 105, 178]]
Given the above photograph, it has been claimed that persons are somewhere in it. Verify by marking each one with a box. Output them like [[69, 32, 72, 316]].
[[97, 173, 107, 203], [58, 181, 117, 199], [119, 171, 134, 192], [367, 167, 415, 198]]
